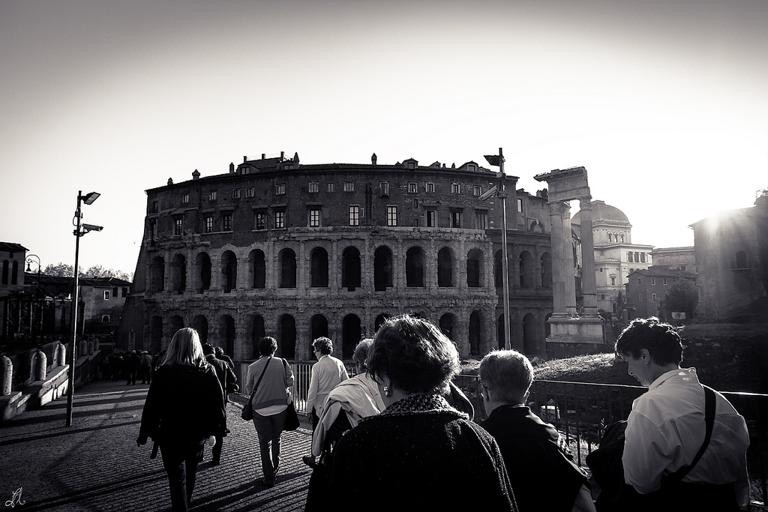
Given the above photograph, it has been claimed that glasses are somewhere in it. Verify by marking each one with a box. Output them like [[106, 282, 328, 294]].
[[363, 358, 385, 386], [312, 350, 317, 354]]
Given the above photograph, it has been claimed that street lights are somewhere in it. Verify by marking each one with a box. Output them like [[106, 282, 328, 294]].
[[483, 149, 512, 350], [65, 190, 101, 425]]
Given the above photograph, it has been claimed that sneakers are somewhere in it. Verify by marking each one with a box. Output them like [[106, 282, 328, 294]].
[[302, 456, 314, 468]]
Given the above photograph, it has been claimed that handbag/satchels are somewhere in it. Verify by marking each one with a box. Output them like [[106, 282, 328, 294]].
[[241, 404, 253, 422], [283, 401, 299, 432]]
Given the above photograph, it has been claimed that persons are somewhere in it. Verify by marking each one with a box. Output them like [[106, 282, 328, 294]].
[[100, 343, 239, 464], [478, 349, 595, 512], [302, 316, 518, 512], [246, 337, 294, 487], [615, 316, 751, 512], [135, 326, 226, 512]]
